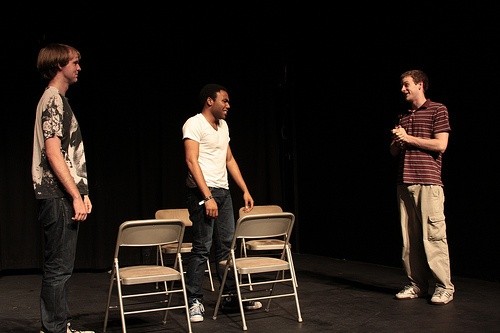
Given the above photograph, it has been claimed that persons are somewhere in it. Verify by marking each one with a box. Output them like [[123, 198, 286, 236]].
[[182, 84, 263, 323], [390, 70, 455, 305], [32, 44, 96, 333]]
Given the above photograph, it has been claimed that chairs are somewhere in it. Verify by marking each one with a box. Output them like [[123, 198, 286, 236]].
[[155, 209, 215, 295], [239, 205, 298, 291], [105, 219, 192, 333], [213, 213, 303, 331]]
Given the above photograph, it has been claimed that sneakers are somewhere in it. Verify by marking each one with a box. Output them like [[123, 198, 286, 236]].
[[223, 296, 263, 309], [431, 288, 453, 305], [189, 298, 205, 322], [395, 285, 418, 298]]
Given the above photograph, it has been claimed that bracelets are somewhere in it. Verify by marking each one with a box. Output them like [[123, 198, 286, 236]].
[[198, 195, 213, 206]]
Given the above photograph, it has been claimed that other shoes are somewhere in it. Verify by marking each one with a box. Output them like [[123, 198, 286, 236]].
[[40, 322, 95, 333]]
